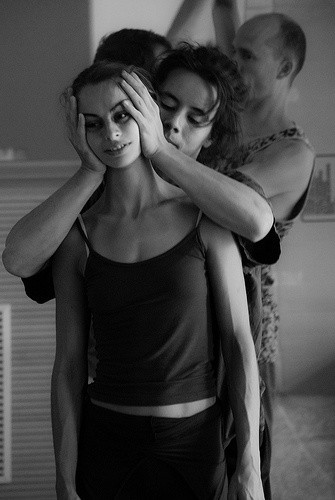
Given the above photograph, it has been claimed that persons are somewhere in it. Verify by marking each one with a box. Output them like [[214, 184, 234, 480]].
[[0, 38, 284, 302], [84, 26, 174, 77], [37, 59, 272, 500], [194, 10, 316, 497]]
